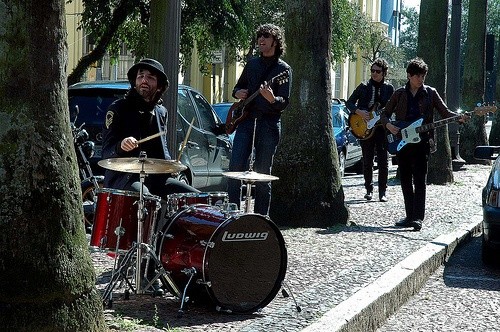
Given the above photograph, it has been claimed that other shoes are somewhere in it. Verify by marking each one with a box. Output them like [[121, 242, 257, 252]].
[[413, 219, 423, 230], [141, 278, 159, 293], [364, 188, 373, 200], [395, 218, 415, 227], [379, 190, 388, 202]]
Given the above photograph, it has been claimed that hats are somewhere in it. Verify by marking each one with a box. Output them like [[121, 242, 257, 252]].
[[127, 58, 168, 91]]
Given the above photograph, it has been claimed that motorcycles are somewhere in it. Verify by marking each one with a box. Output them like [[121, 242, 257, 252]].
[[69, 104, 106, 235]]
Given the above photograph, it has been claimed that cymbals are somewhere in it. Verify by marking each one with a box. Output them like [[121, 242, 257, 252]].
[[97, 157, 188, 174], [220, 170, 280, 181]]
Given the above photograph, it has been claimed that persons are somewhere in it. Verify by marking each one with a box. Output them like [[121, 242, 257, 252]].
[[346, 58, 394, 202], [227, 24, 293, 215], [381, 59, 468, 231], [101, 58, 201, 202]]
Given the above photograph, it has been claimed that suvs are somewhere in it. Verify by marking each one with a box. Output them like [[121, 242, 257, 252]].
[[332, 98, 365, 179]]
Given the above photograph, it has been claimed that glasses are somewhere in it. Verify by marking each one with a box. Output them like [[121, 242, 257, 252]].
[[257, 32, 277, 41], [371, 69, 383, 74]]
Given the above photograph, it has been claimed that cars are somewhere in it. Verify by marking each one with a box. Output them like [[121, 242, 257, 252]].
[[211, 102, 255, 168], [68, 80, 232, 193], [472, 145, 500, 260]]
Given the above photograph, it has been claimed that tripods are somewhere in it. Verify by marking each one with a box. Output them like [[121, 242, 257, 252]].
[[103, 161, 188, 308]]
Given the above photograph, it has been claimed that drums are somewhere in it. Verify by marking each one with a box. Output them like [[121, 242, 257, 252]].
[[158, 203, 288, 316], [90, 188, 162, 250], [167, 191, 229, 218]]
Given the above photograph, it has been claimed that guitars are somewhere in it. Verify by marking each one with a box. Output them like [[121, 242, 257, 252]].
[[224, 70, 290, 135], [347, 101, 386, 141], [382, 101, 497, 155]]
